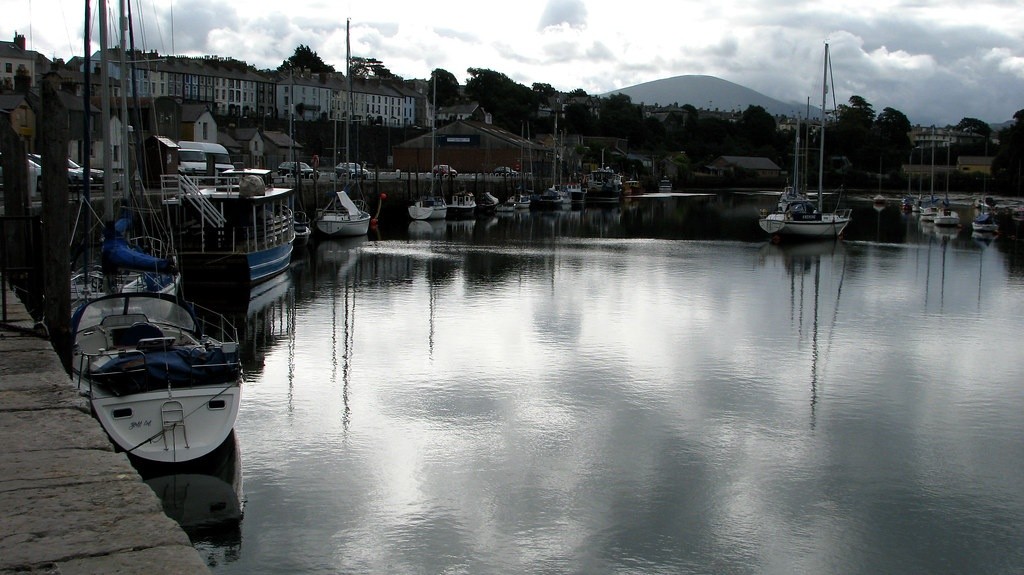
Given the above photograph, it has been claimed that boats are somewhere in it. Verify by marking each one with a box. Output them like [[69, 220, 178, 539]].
[[408, 71, 645, 221], [127, 434, 246, 534], [922, 191, 1024, 232], [658, 176, 674, 193]]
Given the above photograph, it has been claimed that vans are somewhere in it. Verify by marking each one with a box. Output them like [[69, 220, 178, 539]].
[[178, 140, 234, 185]]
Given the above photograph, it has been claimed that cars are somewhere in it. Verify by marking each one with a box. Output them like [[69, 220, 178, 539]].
[[334, 162, 371, 181], [493, 166, 520, 179], [432, 165, 458, 178], [31, 153, 105, 188], [277, 160, 320, 180]]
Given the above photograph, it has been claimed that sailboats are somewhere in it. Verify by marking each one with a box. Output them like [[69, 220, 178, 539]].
[[972, 142, 1000, 232], [758, 41, 853, 240], [934, 131, 961, 226], [33, 0, 386, 466], [873, 155, 887, 204], [912, 140, 925, 212], [900, 150, 915, 210], [918, 136, 942, 223]]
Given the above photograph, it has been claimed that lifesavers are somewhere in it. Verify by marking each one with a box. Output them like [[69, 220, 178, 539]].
[[773, 236, 780, 243], [312, 155, 319, 167]]
